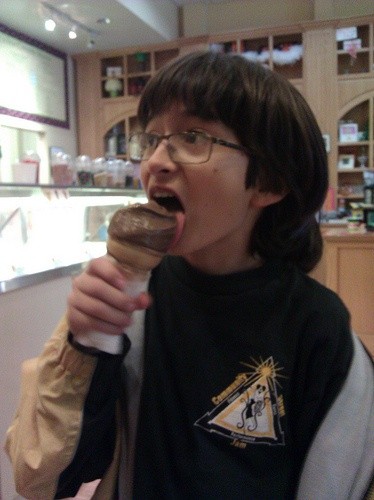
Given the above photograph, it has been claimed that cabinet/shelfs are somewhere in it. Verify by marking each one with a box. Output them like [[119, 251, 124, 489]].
[[72, 15, 374, 359]]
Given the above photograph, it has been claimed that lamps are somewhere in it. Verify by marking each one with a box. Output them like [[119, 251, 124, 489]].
[[44, 12, 98, 49]]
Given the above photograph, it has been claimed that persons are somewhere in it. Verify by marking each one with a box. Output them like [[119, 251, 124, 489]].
[[3, 49, 374, 500]]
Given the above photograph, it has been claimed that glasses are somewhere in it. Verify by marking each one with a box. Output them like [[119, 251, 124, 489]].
[[127, 128, 245, 166]]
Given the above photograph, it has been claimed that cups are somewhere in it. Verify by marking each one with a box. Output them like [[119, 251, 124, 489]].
[[52, 153, 133, 188], [12, 162, 37, 183]]
